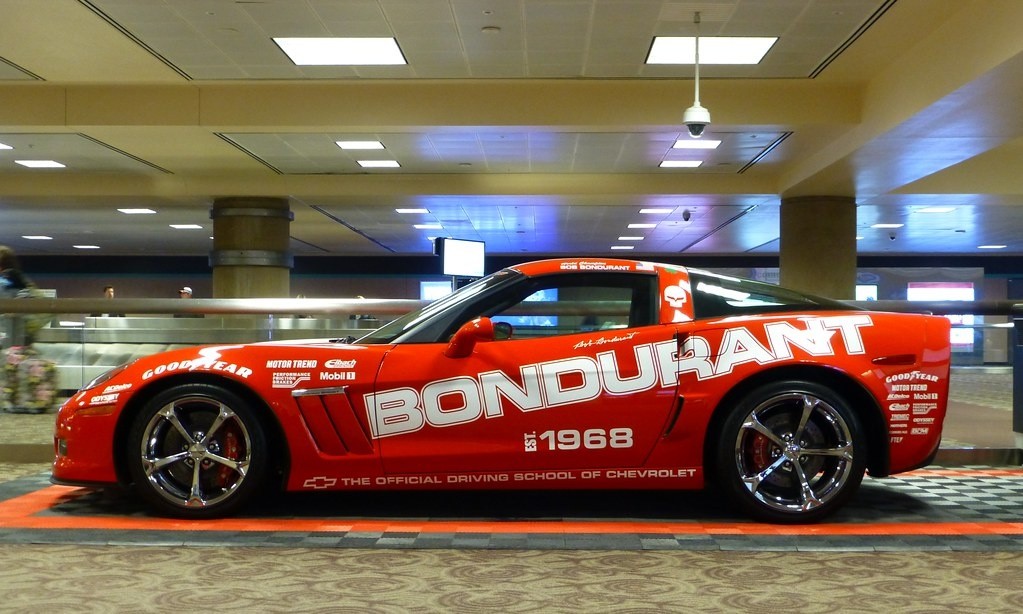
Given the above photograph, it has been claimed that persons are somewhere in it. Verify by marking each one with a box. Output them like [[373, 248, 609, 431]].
[[173, 286, 205, 318], [348, 296, 375, 319], [292, 295, 313, 319], [0, 245, 55, 342], [89, 285, 125, 317]]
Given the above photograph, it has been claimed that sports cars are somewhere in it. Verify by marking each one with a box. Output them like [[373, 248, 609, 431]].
[[46, 255, 954, 522]]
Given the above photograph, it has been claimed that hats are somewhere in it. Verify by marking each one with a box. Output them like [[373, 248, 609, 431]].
[[179, 287, 193, 295]]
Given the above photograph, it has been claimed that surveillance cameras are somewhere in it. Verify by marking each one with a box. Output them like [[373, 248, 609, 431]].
[[681, 108, 711, 137], [683, 213, 690, 221], [889, 233, 896, 240]]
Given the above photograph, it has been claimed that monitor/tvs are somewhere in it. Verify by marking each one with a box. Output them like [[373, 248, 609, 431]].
[[439, 237, 486, 278], [419, 281, 451, 300]]
